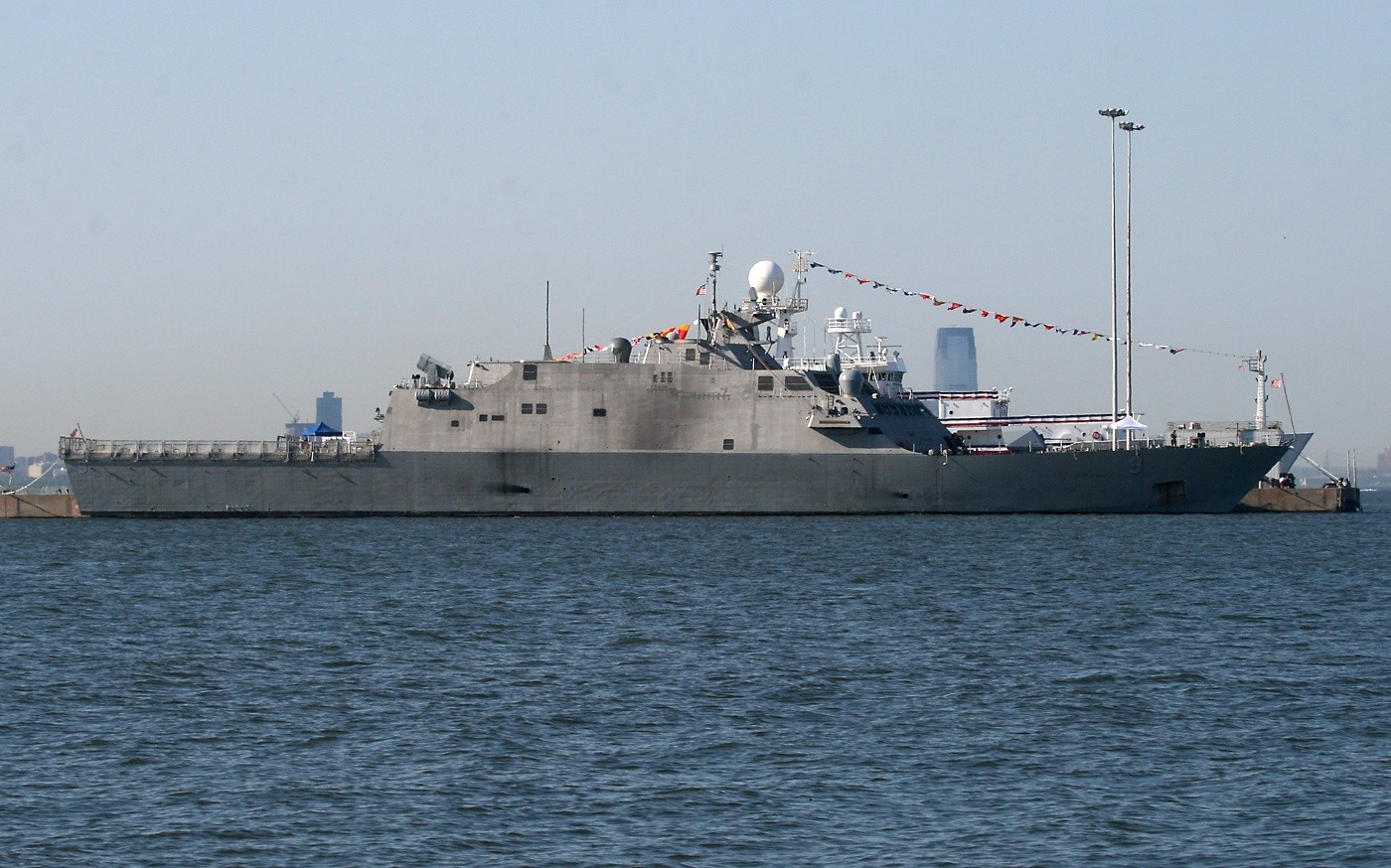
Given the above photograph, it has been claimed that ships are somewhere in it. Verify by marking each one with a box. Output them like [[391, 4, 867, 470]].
[[54, 242, 1293, 516], [913, 383, 1316, 484]]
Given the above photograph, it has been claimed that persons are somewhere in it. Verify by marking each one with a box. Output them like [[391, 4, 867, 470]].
[[1170, 429, 1178, 449], [868, 351, 876, 364]]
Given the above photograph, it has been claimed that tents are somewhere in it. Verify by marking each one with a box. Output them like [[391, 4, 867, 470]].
[[1111, 415, 1148, 432]]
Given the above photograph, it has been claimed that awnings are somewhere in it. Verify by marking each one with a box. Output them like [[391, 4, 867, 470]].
[[301, 421, 342, 437]]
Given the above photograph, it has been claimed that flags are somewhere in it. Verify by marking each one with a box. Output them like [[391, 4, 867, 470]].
[[1271, 379, 1281, 389], [696, 283, 707, 296]]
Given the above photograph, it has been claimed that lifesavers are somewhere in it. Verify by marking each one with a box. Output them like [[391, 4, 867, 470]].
[[1092, 432, 1100, 439]]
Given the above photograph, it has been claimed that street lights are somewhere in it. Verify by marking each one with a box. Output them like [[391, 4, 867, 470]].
[[1115, 120, 1146, 444], [1098, 107, 1130, 450]]
